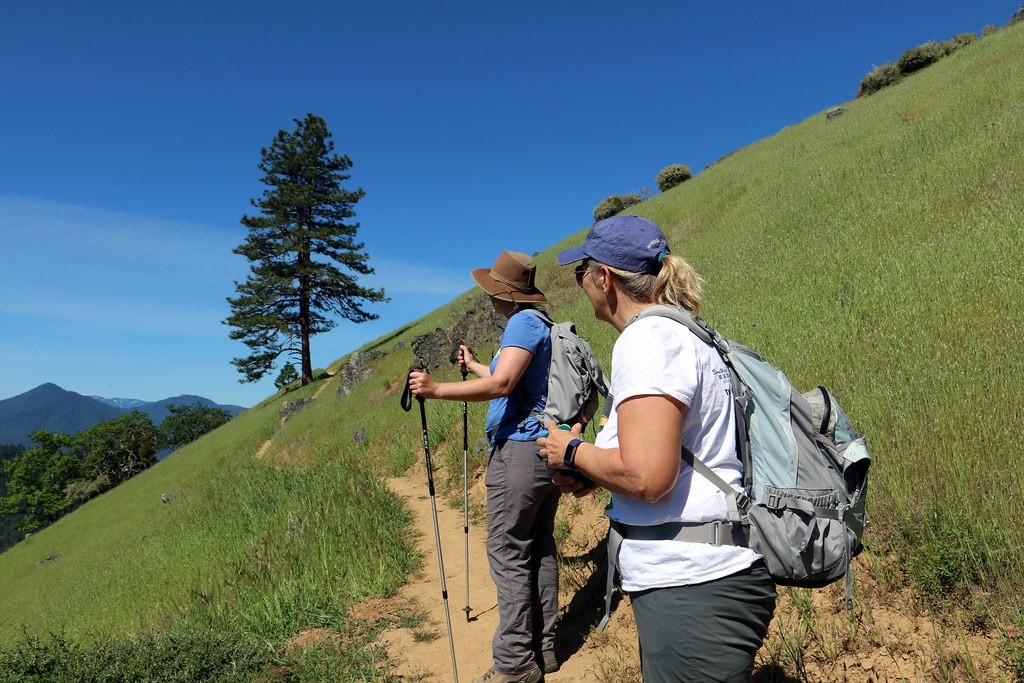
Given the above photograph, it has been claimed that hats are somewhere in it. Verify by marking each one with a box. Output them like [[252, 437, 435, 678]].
[[556, 215, 671, 276], [470, 250, 548, 304]]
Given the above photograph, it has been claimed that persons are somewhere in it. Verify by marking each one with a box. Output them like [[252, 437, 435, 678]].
[[534, 214, 777, 683], [409, 248, 576, 683]]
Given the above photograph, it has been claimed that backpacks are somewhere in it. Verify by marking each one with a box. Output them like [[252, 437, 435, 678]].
[[620, 308, 872, 587], [526, 309, 609, 431]]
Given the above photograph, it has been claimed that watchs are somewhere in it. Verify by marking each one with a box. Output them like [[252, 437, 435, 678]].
[[564, 437, 585, 469]]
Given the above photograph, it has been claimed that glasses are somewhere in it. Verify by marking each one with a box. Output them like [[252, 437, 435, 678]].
[[574, 265, 601, 289]]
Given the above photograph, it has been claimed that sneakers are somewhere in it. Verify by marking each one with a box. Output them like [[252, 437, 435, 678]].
[[472, 662, 541, 683], [536, 650, 559, 674]]
